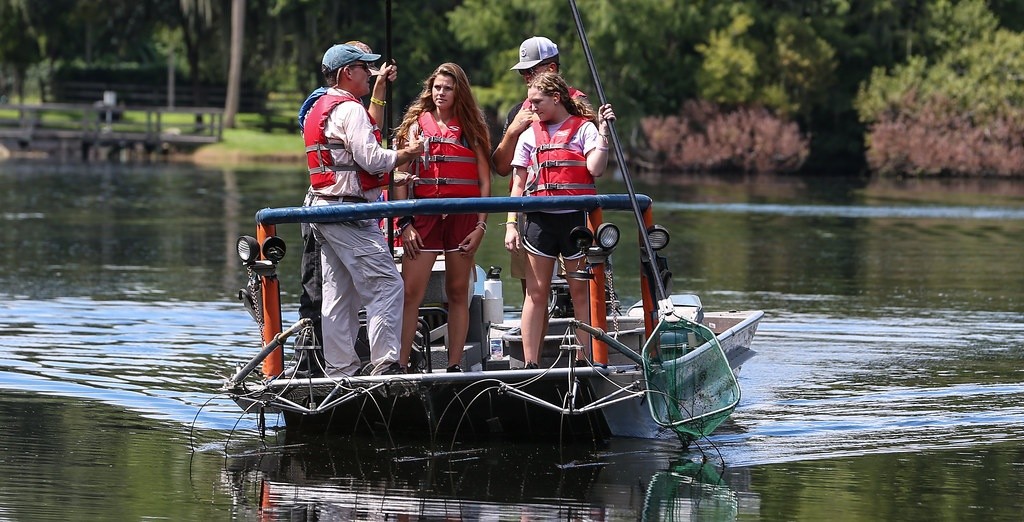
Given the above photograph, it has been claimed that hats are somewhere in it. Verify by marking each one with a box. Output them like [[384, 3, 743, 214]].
[[345, 40, 383, 75], [510, 35, 559, 69], [322, 44, 381, 74]]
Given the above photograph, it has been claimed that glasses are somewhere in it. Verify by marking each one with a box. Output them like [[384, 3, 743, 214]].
[[519, 60, 561, 76], [342, 62, 369, 72]]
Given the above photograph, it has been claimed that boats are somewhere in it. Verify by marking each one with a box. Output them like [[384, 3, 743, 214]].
[[234, 193, 766, 441]]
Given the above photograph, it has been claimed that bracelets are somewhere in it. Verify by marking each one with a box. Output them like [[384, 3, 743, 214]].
[[396, 216, 415, 230], [506, 217, 518, 224], [599, 134, 610, 136], [370, 98, 386, 106], [475, 222, 487, 233], [596, 146, 609, 151]]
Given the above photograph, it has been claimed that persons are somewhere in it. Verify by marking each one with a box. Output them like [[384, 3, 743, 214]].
[[505, 73, 616, 369], [393, 62, 492, 374], [294, 41, 424, 378], [491, 36, 597, 365]]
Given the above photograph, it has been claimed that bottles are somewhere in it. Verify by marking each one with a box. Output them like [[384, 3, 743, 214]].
[[483, 265, 503, 299]]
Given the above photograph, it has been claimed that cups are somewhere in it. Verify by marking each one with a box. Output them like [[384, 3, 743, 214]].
[[482, 298, 503, 324]]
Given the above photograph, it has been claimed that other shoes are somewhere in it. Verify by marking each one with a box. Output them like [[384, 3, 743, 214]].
[[575, 359, 588, 367], [382, 363, 405, 374], [524, 360, 538, 369], [447, 364, 461, 372]]
